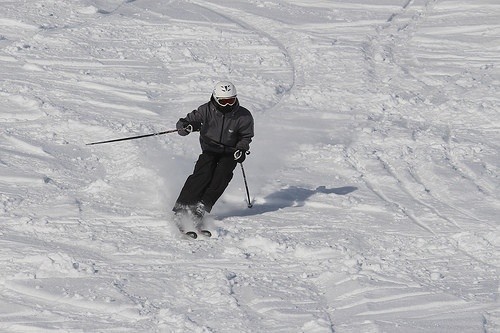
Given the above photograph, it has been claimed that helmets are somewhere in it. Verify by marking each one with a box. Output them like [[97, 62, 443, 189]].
[[213, 81, 238, 107]]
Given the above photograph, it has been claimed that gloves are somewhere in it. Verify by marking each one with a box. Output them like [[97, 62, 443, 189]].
[[177, 124, 192, 137], [234, 149, 247, 163]]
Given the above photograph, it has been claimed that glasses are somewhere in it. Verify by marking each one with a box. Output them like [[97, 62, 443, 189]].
[[216, 98, 236, 107]]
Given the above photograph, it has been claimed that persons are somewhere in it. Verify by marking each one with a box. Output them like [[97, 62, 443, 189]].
[[171, 81, 255, 227]]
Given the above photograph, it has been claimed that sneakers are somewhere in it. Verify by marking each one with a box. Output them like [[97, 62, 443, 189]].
[[174, 209, 190, 224], [191, 201, 207, 223]]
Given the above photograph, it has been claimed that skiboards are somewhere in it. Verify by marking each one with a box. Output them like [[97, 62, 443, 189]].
[[171, 200, 213, 239]]
